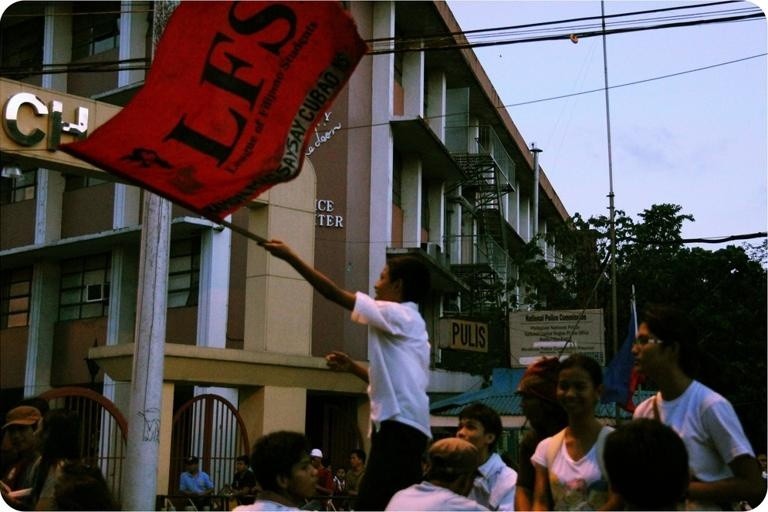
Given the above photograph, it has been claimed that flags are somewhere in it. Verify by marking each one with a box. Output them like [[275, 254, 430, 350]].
[[58, 2, 372, 226]]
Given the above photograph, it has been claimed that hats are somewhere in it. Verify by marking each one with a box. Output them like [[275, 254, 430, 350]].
[[309, 449, 322, 458], [1, 406, 41, 430], [186, 457, 198, 464], [514, 374, 554, 400], [420, 438, 483, 482]]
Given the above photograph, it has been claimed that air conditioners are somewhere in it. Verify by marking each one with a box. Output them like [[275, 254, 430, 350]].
[[84, 282, 113, 303]]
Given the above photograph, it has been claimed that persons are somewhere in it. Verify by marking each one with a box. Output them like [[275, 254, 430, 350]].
[[1, 397, 117, 510], [511, 306, 767, 511], [383, 403, 517, 511], [256, 237, 436, 511], [179, 430, 366, 511]]
